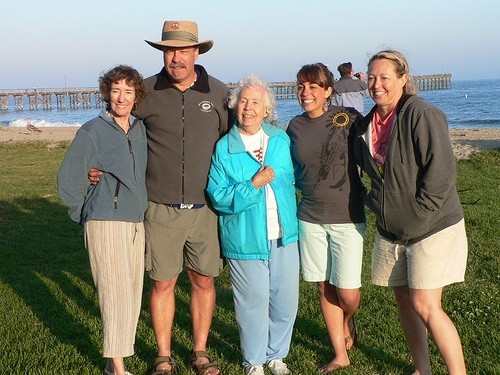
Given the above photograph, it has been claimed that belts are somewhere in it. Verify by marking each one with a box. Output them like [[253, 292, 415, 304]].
[[164, 204, 206, 209]]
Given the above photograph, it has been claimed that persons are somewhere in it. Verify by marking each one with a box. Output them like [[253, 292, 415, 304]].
[[285, 63, 364, 374], [87, 21, 235, 375], [352, 50, 468, 375], [57, 66, 148, 375], [205, 74, 300, 375], [333, 62, 368, 115]]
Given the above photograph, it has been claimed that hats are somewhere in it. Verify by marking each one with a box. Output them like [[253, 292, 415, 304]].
[[144, 21, 214, 54]]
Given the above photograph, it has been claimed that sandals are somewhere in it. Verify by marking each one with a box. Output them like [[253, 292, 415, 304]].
[[188, 351, 221, 375], [152, 356, 173, 375]]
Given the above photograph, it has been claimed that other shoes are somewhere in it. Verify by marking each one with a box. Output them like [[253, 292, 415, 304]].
[[104, 368, 132, 375]]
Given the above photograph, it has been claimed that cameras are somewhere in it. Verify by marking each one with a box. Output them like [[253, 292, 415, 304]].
[[354, 73, 360, 77]]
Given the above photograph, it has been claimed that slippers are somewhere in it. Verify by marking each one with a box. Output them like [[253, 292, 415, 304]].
[[318, 362, 350, 375], [344, 316, 356, 351]]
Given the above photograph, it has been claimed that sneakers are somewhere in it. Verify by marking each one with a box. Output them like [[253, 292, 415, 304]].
[[268, 360, 291, 375], [243, 362, 265, 375]]
